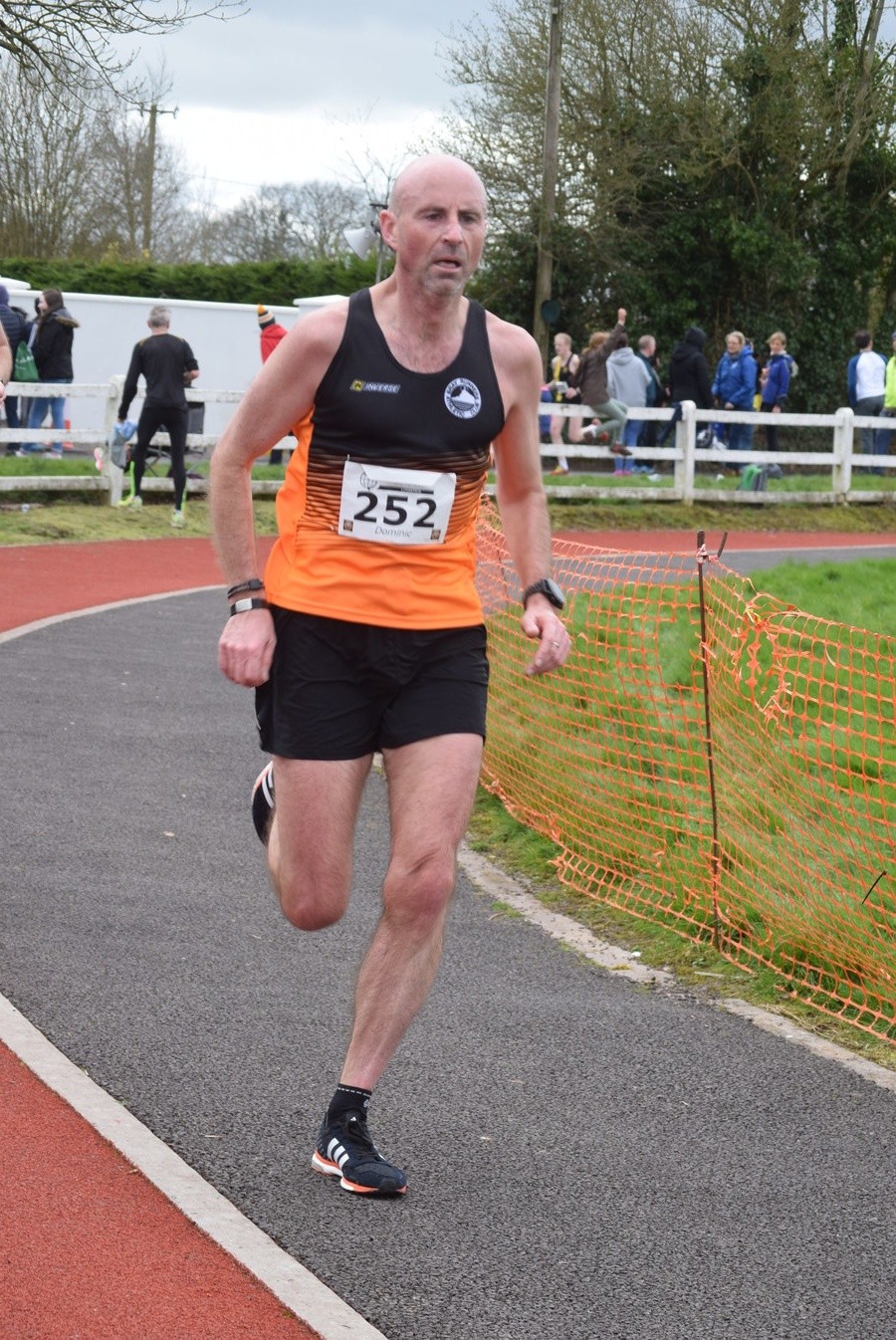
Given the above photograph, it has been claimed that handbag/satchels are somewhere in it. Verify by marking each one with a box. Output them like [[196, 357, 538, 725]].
[[14, 341, 40, 382]]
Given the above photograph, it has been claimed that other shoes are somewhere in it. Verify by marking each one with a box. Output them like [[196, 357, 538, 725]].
[[16, 451, 26, 458], [584, 431, 595, 444], [614, 470, 623, 476], [624, 470, 632, 476], [549, 466, 569, 475], [610, 443, 632, 455], [592, 418, 610, 442], [45, 452, 61, 459]]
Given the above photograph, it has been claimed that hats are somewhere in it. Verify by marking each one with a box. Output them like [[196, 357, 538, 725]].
[[257, 305, 273, 324]]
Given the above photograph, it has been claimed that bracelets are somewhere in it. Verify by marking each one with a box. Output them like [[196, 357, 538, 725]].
[[227, 579, 265, 598], [230, 597, 269, 616]]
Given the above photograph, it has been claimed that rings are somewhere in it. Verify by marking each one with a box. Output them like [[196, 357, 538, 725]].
[[552, 641, 559, 648]]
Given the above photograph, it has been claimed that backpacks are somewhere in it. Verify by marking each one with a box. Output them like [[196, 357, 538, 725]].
[[736, 465, 766, 508]]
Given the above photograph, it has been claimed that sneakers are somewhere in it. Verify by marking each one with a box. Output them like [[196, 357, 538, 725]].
[[251, 761, 276, 849], [311, 1108, 407, 1196], [116, 496, 142, 512], [170, 510, 186, 527]]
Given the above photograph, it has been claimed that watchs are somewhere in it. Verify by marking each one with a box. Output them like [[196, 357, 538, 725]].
[[522, 578, 566, 611]]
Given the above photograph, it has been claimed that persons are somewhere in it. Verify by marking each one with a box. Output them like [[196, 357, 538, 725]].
[[545, 308, 791, 483], [847, 329, 896, 477], [257, 303, 295, 466], [117, 307, 199, 529], [211, 152, 571, 1197], [0, 284, 80, 460]]
